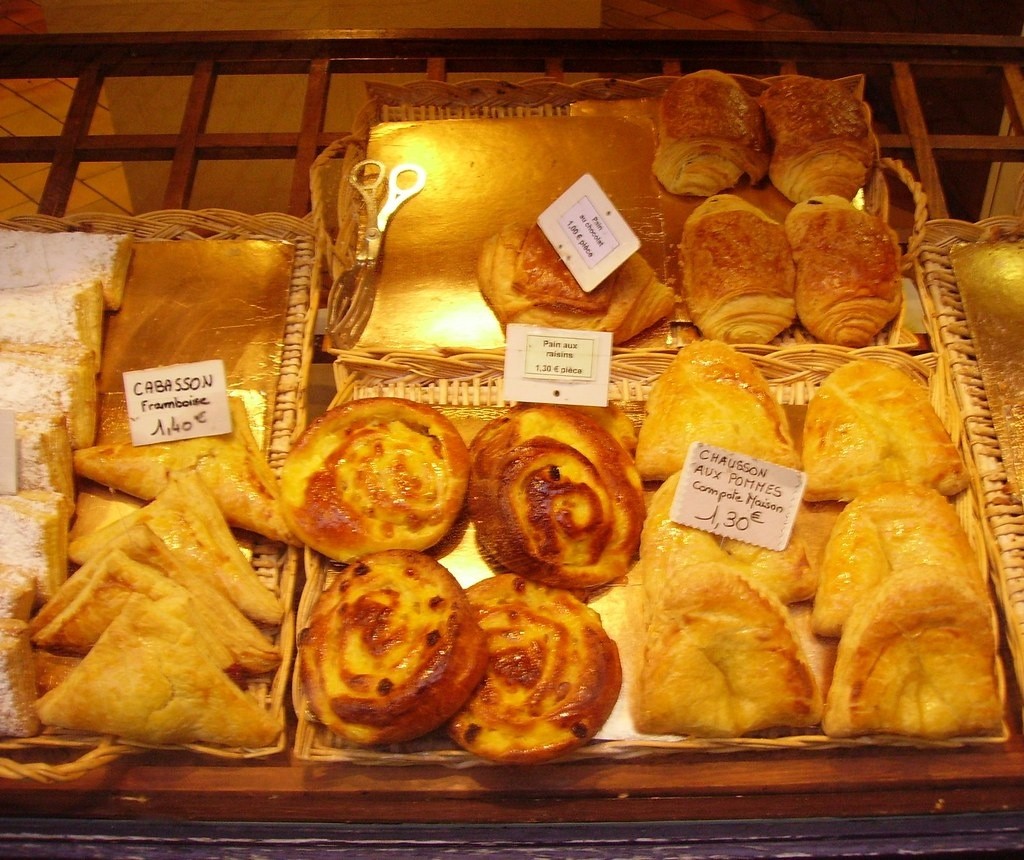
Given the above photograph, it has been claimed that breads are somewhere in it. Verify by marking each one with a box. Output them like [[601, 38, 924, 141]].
[[0, 70, 1001, 763]]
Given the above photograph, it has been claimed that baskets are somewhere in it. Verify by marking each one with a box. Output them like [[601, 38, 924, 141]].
[[0, 73, 1024, 785]]
